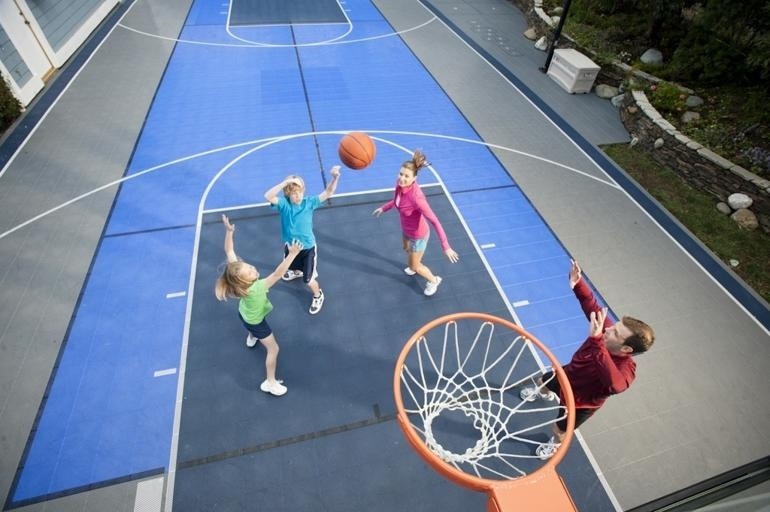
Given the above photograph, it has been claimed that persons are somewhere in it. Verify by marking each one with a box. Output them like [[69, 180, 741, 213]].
[[371, 148, 459, 296], [521, 257, 657, 461], [263, 165, 349, 316], [214, 213, 305, 398]]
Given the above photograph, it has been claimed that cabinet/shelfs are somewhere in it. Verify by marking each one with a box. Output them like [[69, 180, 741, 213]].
[[546, 47, 601, 95]]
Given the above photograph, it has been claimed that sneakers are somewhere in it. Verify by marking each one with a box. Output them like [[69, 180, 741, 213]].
[[260, 379, 288, 396], [282, 269, 303, 281], [424, 275, 442, 296], [535, 436, 561, 461], [404, 267, 416, 276], [520, 387, 555, 402], [246, 333, 258, 347], [309, 289, 325, 314]]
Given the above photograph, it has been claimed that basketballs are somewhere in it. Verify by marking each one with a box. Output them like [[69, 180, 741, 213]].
[[338, 133, 376, 169]]
